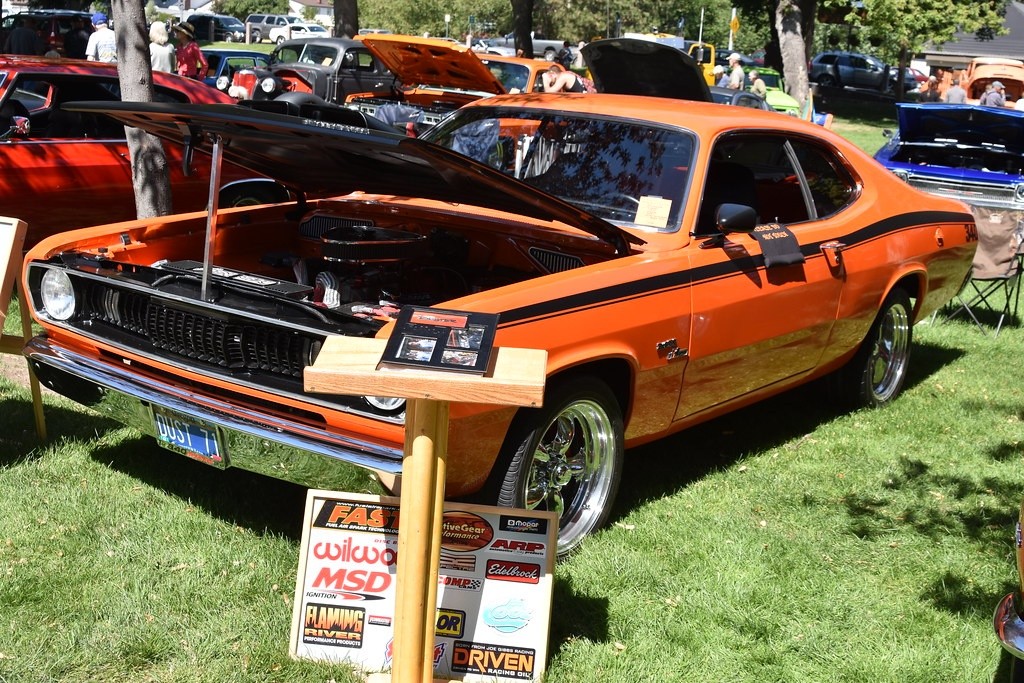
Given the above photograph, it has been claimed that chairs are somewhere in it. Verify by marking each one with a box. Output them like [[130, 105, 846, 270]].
[[0, 216, 48, 448], [931, 203, 1024, 340]]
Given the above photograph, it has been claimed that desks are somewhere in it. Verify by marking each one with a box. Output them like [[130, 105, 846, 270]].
[[302, 333, 549, 683]]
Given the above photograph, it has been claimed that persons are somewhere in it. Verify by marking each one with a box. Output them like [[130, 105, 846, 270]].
[[85, 11, 119, 63], [171, 22, 208, 81], [63, 13, 89, 59], [406, 351, 431, 361], [712, 65, 731, 88], [558, 41, 573, 71], [148, 20, 176, 74], [409, 340, 437, 348], [980, 81, 1006, 106], [748, 69, 766, 109], [460, 333, 482, 350], [44, 44, 61, 58], [944, 79, 968, 104], [920, 82, 943, 101], [514, 47, 529, 80], [929, 69, 944, 96], [541, 65, 586, 93], [1014, 93, 1024, 110], [3, 20, 37, 55], [726, 52, 745, 91]]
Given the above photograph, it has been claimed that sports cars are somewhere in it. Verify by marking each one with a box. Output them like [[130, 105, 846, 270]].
[[21, 92, 980, 559], [0, 10, 1024, 218]]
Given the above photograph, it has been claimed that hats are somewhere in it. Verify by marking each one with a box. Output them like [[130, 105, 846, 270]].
[[712, 65, 725, 75], [992, 81, 1006, 89], [726, 53, 742, 61], [929, 76, 939, 83], [91, 13, 107, 25], [171, 22, 195, 38]]
